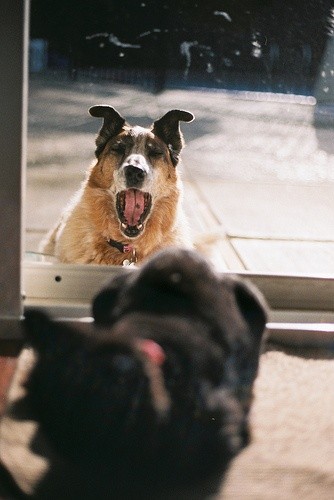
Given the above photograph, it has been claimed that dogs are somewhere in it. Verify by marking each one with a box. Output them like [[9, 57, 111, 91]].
[[0, 242, 271, 500], [40, 105, 196, 270]]
